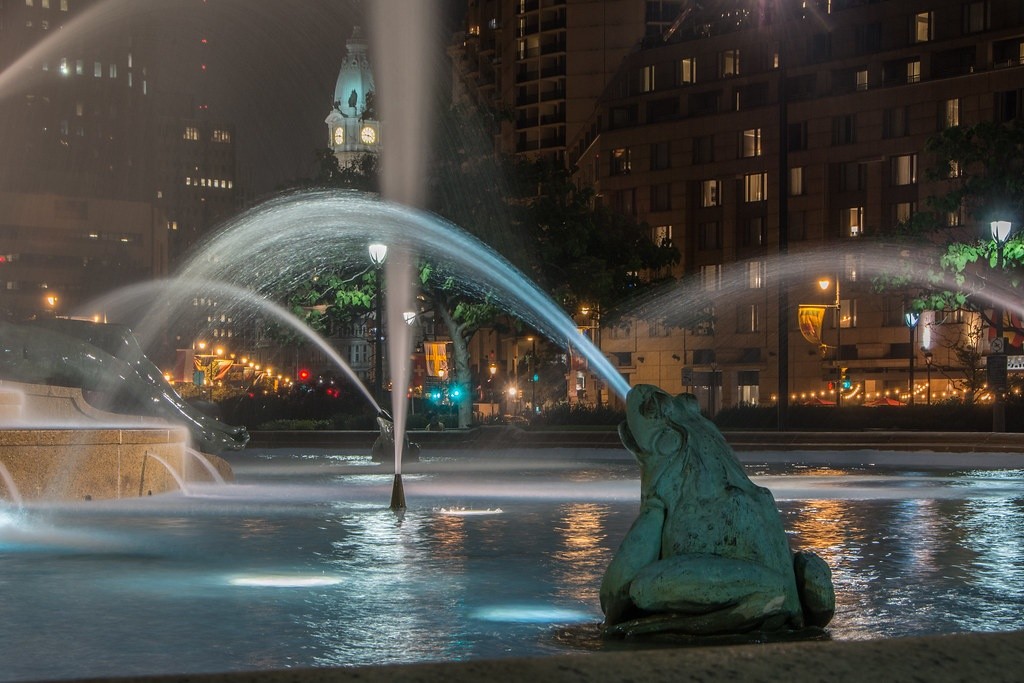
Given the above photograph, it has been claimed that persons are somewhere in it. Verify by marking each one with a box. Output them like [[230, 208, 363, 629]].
[[425, 410, 445, 431]]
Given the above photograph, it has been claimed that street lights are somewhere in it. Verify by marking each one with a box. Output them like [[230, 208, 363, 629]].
[[368, 242, 390, 402], [904, 309, 921, 408], [528, 333, 537, 420], [925, 351, 936, 404], [818, 253, 846, 409], [988, 212, 1015, 353], [199, 342, 223, 402]]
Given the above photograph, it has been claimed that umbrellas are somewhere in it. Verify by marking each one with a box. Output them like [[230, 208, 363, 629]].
[[803, 397, 836, 406], [869, 396, 907, 406]]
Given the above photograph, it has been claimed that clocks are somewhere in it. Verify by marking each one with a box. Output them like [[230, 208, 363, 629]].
[[334, 125, 345, 145], [360, 125, 376, 145]]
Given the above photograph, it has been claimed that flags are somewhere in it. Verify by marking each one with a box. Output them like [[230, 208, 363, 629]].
[[424, 344, 448, 380]]
